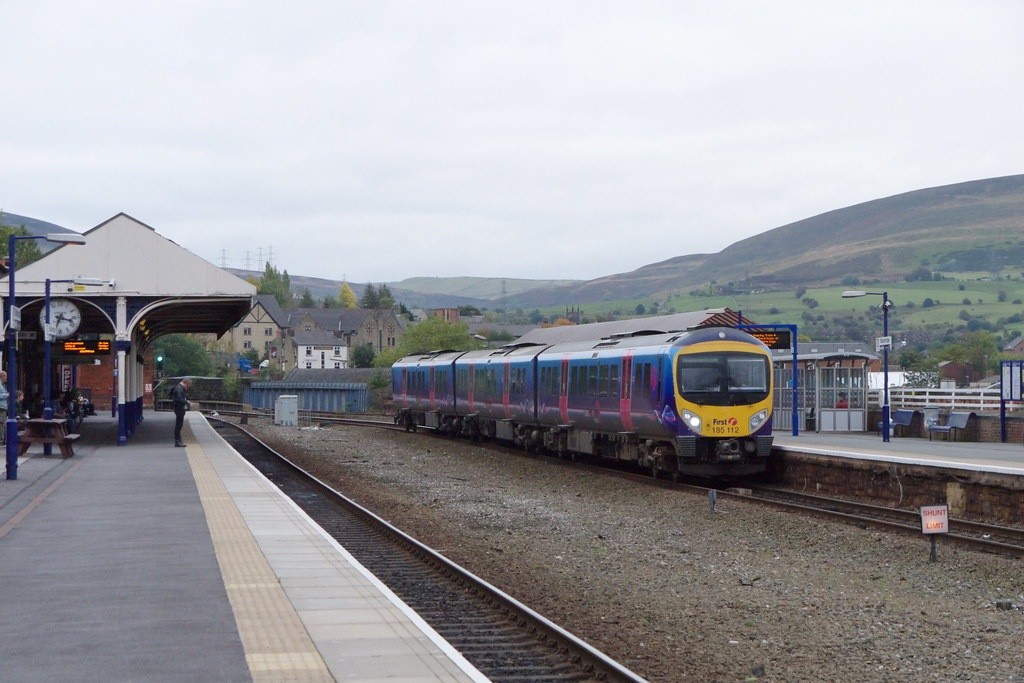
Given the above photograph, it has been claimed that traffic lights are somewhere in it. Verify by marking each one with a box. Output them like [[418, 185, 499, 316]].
[[157, 356, 163, 370]]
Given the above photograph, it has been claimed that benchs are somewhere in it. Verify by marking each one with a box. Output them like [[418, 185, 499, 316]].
[[17, 417, 82, 460], [878, 409, 921, 438], [926, 411, 978, 442]]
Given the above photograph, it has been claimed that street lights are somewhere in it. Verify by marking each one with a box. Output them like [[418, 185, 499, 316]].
[[6, 233, 87, 481], [841, 290, 891, 442], [43, 278, 104, 455]]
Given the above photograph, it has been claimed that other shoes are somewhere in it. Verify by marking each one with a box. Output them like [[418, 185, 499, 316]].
[[176, 440, 186, 447]]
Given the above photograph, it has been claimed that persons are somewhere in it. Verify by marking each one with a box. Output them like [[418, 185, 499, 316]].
[[711, 368, 738, 387], [835, 392, 848, 408], [0, 370, 10, 446], [14, 389, 98, 432], [171, 378, 193, 448]]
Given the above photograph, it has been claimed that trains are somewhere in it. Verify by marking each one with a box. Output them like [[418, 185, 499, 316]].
[[389, 327, 773, 483]]
